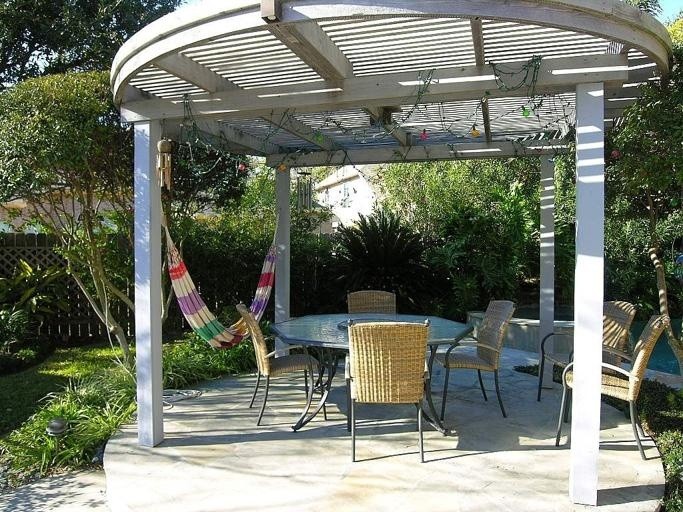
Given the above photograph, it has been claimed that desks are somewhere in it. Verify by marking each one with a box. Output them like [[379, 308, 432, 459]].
[[269, 311, 474, 435]]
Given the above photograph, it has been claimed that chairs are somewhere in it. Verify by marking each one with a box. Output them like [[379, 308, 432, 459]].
[[235, 303, 327, 426], [555, 314, 671, 461], [436, 301, 517, 422], [536, 300, 637, 401], [343, 319, 430, 463], [347, 292, 397, 315]]
